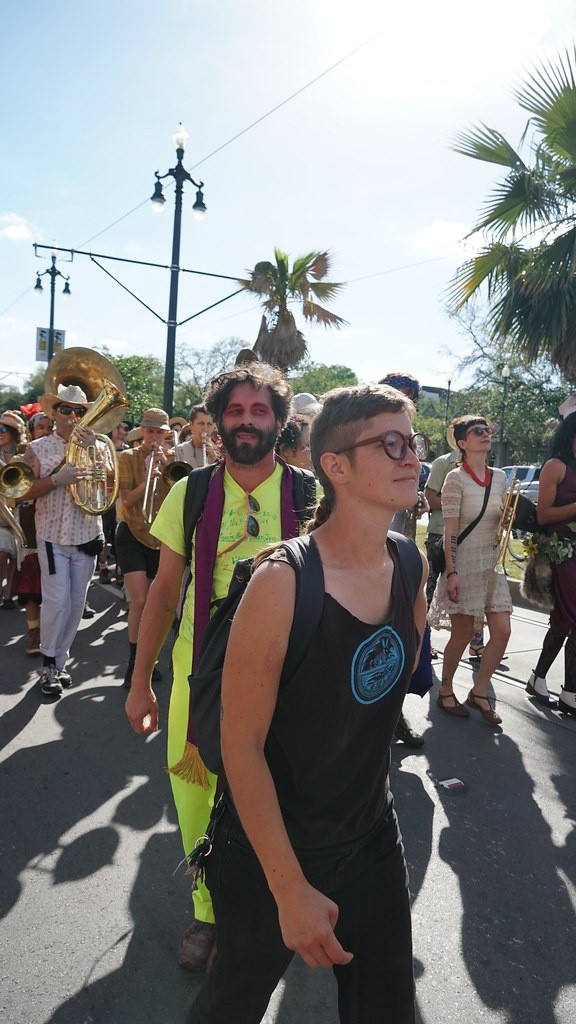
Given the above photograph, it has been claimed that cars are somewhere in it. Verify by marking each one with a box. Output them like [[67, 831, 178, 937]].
[[418, 461, 431, 492]]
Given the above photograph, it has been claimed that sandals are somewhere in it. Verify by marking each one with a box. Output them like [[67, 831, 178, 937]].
[[469, 647, 508, 660], [467, 690, 501, 723], [437, 692, 470, 716]]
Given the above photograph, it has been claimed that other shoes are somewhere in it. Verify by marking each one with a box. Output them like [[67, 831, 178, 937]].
[[4, 598, 16, 610], [526, 672, 557, 708], [81, 566, 126, 616], [558, 690, 576, 716], [392, 718, 425, 747], [24, 628, 42, 652], [83, 609, 95, 619]]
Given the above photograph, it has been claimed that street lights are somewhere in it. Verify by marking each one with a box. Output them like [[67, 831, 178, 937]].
[[498, 364, 511, 468], [33, 248, 71, 366], [149, 120, 208, 419]]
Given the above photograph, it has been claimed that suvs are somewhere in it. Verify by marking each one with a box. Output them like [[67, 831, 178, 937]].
[[500, 465, 542, 506]]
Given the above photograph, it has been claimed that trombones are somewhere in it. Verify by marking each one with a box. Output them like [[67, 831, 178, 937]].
[[0, 461, 37, 550], [141, 431, 194, 525], [200, 431, 208, 466]]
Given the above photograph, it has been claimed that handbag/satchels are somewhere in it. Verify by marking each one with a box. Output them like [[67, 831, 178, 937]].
[[77, 535, 105, 556], [426, 537, 448, 573]]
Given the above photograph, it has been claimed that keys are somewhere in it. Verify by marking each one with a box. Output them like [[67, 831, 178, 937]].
[[173, 834, 213, 891]]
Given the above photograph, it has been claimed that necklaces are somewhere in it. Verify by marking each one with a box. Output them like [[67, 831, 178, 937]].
[[463, 460, 491, 486]]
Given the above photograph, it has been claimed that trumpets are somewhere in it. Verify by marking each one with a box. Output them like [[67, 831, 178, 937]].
[[492, 465, 522, 576]]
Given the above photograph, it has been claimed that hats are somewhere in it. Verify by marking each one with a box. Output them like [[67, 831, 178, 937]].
[[169, 417, 187, 427], [40, 384, 95, 419], [291, 393, 324, 419], [0, 412, 25, 434], [140, 409, 170, 431]]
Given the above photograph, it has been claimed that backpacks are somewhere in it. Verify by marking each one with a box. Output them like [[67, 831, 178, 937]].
[[186, 530, 425, 777]]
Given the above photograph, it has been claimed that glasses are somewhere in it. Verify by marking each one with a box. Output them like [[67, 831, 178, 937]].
[[57, 405, 86, 418], [460, 427, 489, 437], [0, 425, 14, 434], [116, 427, 129, 432], [215, 491, 261, 559], [332, 430, 431, 461]]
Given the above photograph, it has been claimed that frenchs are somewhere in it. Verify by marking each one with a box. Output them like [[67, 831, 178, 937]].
[[42, 347, 128, 517]]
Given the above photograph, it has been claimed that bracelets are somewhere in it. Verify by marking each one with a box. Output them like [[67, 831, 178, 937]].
[[447, 571, 457, 579]]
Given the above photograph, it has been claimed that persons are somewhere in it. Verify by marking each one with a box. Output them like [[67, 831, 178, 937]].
[[525, 411, 576, 713], [1, 375, 426, 748], [426, 415, 514, 724], [424, 419, 508, 660], [183, 385, 433, 1024], [125, 362, 316, 977]]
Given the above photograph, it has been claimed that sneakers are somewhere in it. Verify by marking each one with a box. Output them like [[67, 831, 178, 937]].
[[57, 668, 72, 686], [38, 664, 63, 696], [178, 920, 217, 971]]
